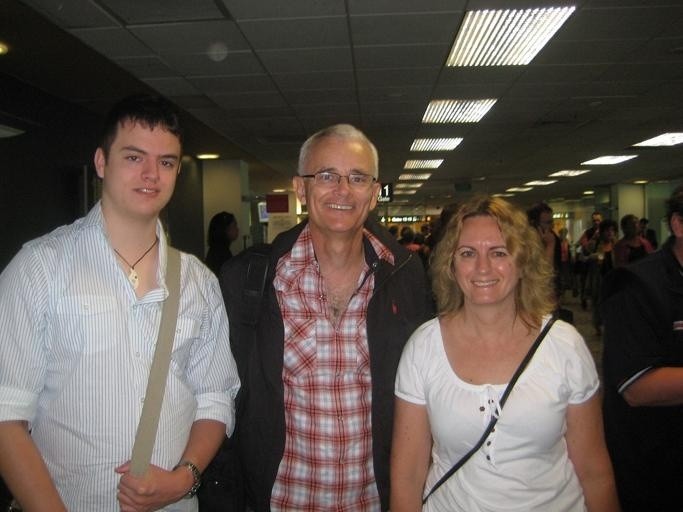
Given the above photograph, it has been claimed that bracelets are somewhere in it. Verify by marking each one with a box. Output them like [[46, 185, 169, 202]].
[[171, 459, 202, 501]]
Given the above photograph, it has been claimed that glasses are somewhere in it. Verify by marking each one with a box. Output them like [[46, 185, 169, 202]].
[[301, 172, 378, 192]]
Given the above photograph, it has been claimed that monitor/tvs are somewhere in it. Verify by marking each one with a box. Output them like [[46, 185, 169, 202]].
[[265, 193, 290, 216]]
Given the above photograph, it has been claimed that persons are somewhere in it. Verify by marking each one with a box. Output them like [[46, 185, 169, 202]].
[[0, 93, 240, 512], [387, 192, 620, 512], [204, 210, 238, 274], [196, 123, 434, 512], [603, 187, 682, 510], [387, 202, 661, 338]]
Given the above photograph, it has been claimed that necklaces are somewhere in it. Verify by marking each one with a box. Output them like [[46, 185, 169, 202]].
[[114, 235, 159, 291]]
